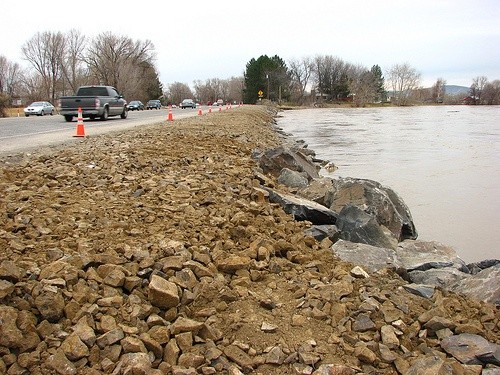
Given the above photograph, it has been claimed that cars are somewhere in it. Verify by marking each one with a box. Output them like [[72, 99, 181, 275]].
[[127, 101, 145, 111], [171, 99, 223, 108], [23, 101, 55, 117], [181, 98, 196, 109]]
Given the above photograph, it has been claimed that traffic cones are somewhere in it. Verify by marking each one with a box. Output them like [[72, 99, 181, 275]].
[[198, 106, 203, 115], [72, 106, 86, 137], [166, 108, 174, 121], [208, 102, 232, 113]]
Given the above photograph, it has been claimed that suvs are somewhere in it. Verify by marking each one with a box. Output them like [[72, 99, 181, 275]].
[[146, 100, 161, 110]]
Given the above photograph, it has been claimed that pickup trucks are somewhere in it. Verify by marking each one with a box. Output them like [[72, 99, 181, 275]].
[[58, 84, 128, 122]]
